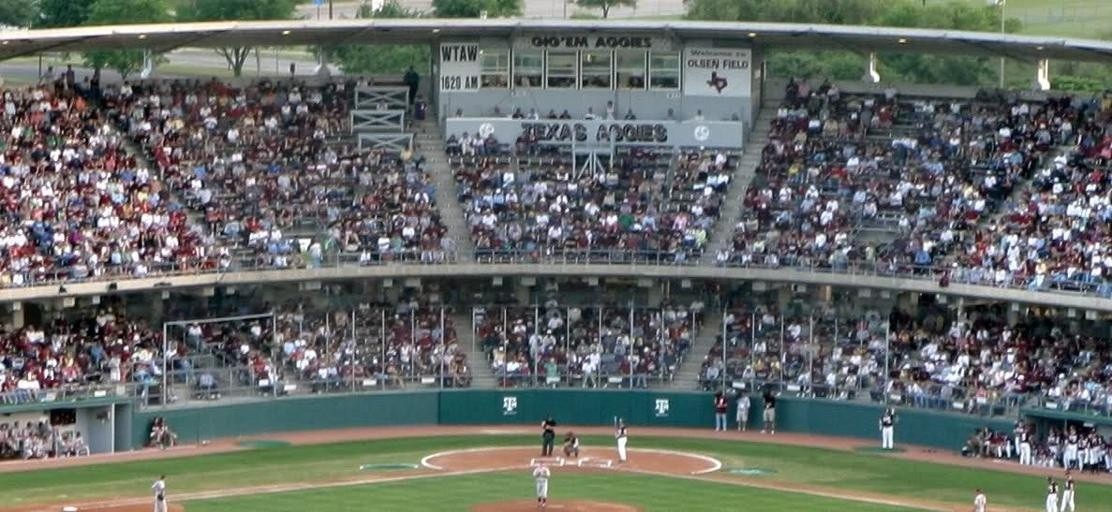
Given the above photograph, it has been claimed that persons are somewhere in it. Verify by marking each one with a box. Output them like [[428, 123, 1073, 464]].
[[1, 61, 1112, 512]]
